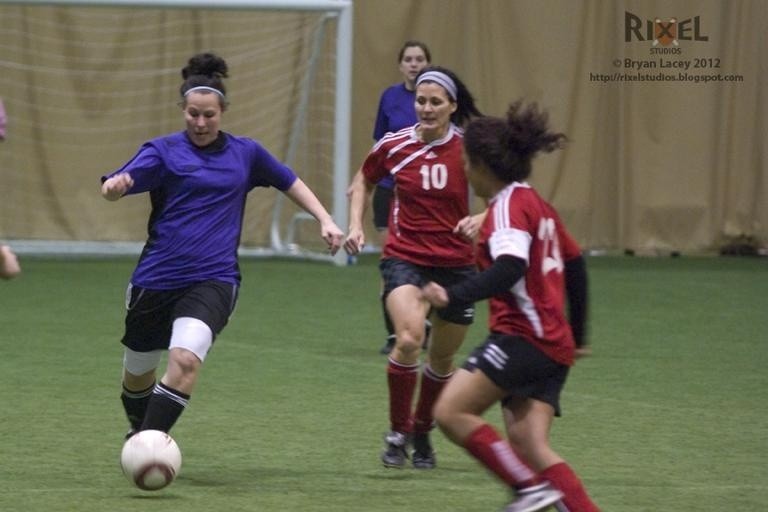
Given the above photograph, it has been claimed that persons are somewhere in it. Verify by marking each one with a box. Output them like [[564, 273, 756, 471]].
[[342, 40, 600, 512], [101, 51, 344, 492], [0, 99, 22, 279]]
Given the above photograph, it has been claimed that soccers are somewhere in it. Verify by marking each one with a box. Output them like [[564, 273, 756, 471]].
[[120, 429, 182, 492]]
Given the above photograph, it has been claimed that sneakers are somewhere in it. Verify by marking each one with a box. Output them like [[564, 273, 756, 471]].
[[501, 478, 566, 512], [410, 419, 439, 468], [381, 428, 409, 468]]
